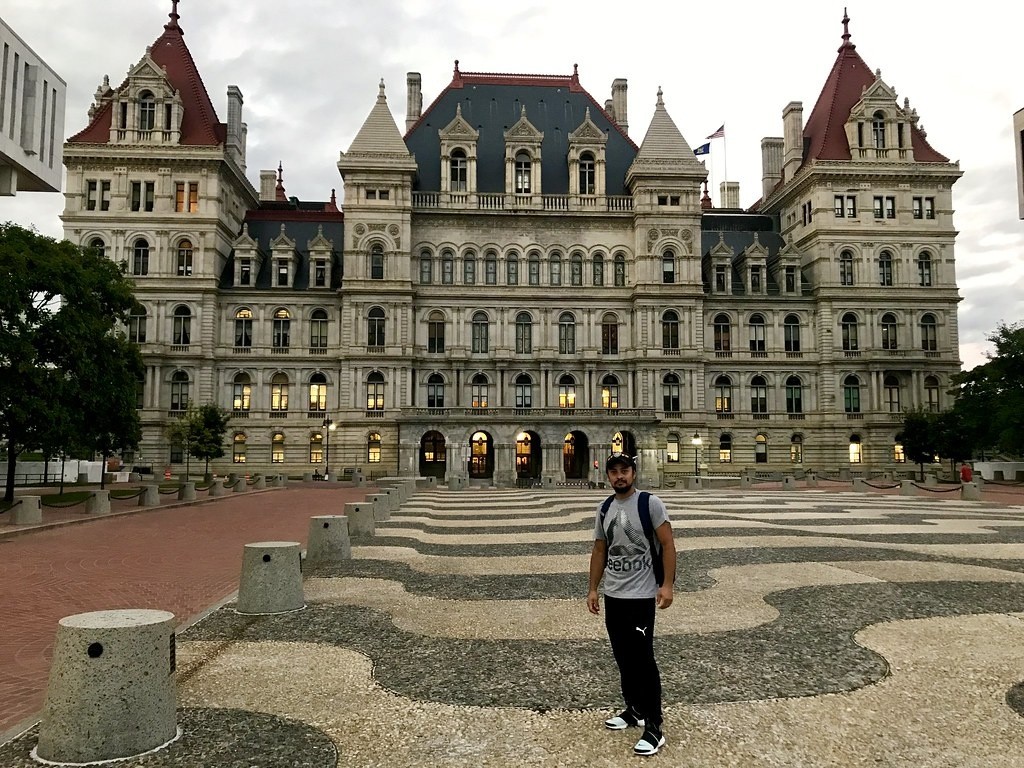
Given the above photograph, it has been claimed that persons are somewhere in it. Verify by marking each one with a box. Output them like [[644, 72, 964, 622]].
[[587, 453, 678, 755], [960, 463, 972, 491]]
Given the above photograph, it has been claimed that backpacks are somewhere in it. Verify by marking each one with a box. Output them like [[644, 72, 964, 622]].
[[601, 492, 676, 587]]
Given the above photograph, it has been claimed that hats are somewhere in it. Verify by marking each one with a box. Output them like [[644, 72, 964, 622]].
[[607, 452, 636, 471]]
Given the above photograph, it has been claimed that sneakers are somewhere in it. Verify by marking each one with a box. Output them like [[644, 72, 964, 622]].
[[633, 722, 665, 755], [604, 706, 645, 729]]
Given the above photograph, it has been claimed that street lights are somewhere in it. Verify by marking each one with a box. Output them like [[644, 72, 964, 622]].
[[322, 414, 333, 481], [691, 429, 702, 483]]
